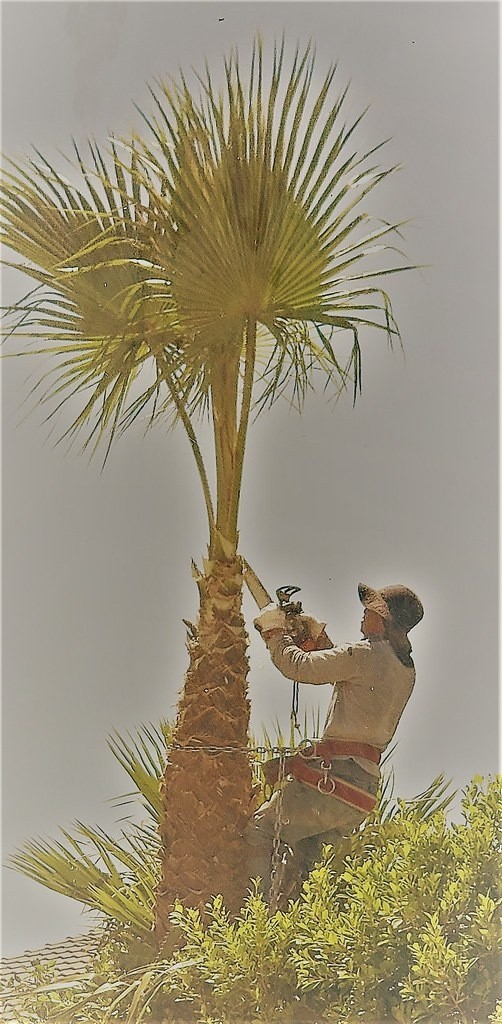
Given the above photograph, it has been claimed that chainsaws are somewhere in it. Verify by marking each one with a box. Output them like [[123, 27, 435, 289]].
[[239, 554, 334, 651]]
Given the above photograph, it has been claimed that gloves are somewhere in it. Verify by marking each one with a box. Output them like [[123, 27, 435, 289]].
[[253, 603, 285, 640], [289, 612, 325, 648]]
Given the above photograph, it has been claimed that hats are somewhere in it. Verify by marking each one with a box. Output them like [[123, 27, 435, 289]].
[[356, 583, 424, 667]]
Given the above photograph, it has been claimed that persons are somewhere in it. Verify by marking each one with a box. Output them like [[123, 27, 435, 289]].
[[246, 581, 424, 915]]
